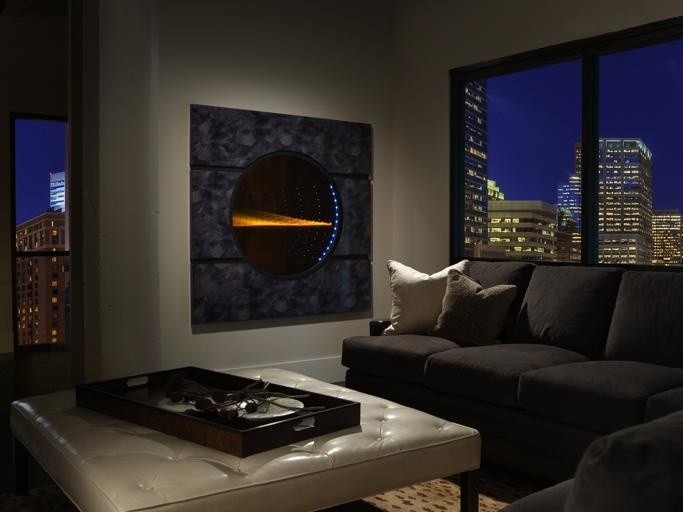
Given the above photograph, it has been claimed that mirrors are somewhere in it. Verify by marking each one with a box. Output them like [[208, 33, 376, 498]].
[[223, 145, 344, 281]]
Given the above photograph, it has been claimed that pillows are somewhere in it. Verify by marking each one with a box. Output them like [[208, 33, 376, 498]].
[[382, 255, 517, 347]]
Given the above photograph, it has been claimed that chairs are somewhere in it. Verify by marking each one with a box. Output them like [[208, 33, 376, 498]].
[[496, 409, 682, 512]]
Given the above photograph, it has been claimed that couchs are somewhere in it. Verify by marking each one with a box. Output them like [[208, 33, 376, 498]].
[[341, 259, 682, 483]]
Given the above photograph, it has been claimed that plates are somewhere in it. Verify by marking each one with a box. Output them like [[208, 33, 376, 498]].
[[232, 395, 305, 422]]
